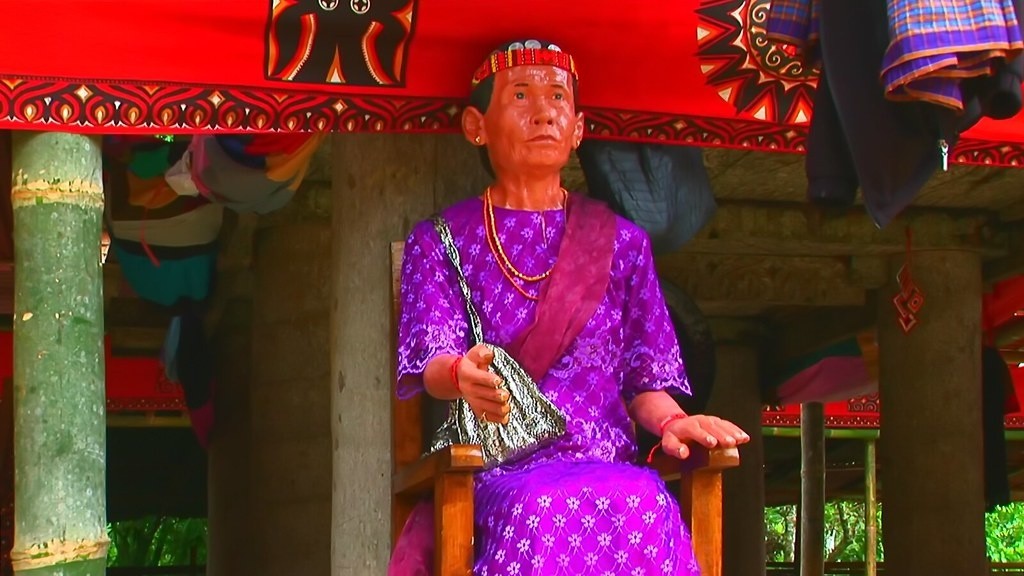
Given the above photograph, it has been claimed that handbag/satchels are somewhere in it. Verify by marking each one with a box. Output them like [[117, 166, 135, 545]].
[[417, 341, 567, 476]]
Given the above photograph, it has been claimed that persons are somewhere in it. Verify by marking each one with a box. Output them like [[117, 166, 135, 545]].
[[395, 38, 750, 576]]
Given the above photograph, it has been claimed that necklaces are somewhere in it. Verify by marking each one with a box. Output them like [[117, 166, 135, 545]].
[[483, 183, 571, 301]]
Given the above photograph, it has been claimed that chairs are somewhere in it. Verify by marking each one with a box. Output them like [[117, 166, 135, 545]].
[[389, 241, 738, 576]]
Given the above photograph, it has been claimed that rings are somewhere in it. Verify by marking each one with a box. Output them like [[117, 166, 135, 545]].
[[479, 411, 491, 424]]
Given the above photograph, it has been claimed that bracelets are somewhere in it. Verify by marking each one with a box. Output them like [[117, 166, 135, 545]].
[[450, 353, 466, 398], [647, 414, 690, 463]]
